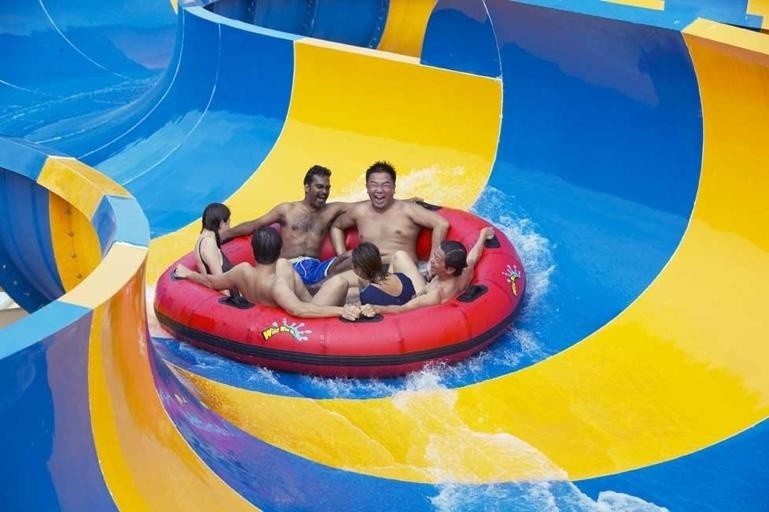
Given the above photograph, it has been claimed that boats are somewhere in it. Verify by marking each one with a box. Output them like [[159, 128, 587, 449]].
[[154, 201, 528, 379]]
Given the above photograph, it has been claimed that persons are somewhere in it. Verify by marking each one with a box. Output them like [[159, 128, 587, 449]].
[[360, 226, 494, 318], [218, 164, 423, 295], [342, 240, 417, 305], [194, 203, 240, 296], [329, 161, 450, 275], [174, 225, 361, 321]]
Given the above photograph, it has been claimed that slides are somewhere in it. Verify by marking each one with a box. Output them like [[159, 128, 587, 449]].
[[1, 0, 769, 512]]
